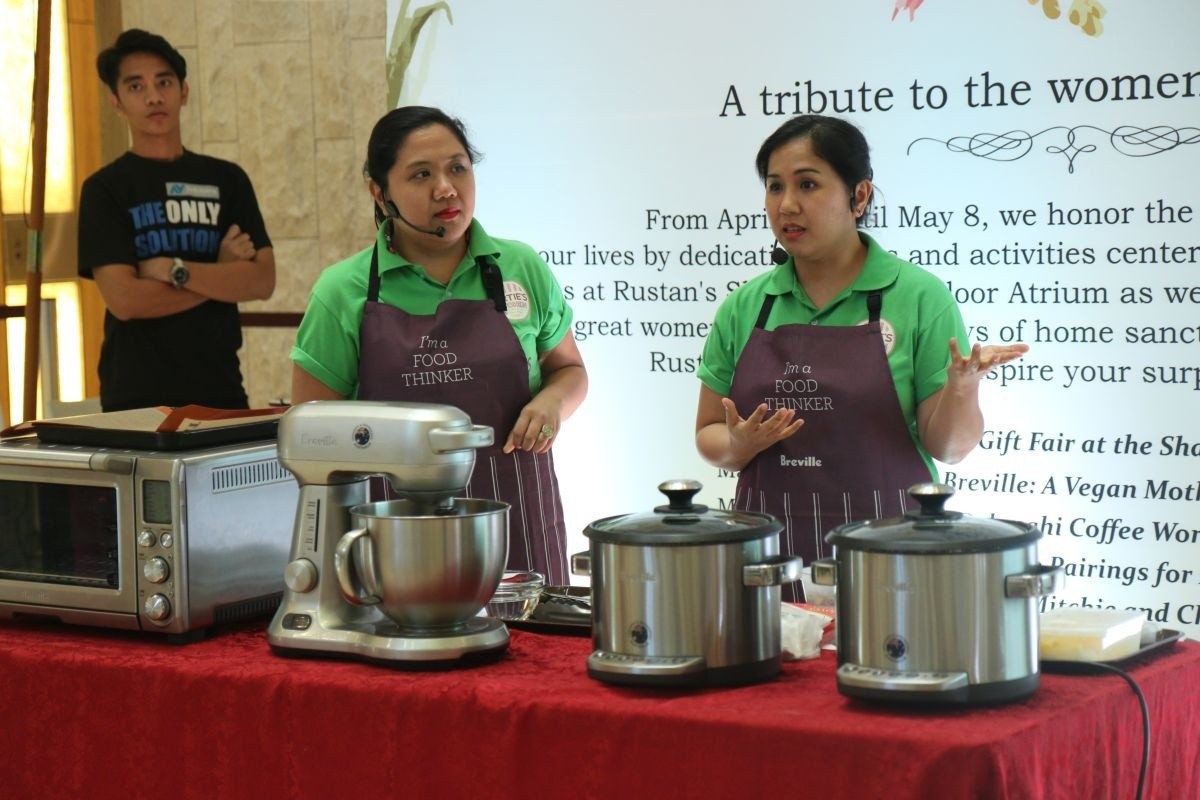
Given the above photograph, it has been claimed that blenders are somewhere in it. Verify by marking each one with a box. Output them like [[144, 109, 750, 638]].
[[265, 390, 517, 666]]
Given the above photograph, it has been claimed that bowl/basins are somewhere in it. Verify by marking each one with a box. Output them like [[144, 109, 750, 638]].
[[482, 568, 545, 622]]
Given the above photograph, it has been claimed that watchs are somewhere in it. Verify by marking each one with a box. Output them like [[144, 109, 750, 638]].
[[168, 259, 189, 291]]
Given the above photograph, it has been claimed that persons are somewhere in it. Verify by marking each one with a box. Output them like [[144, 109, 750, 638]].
[[695, 113, 1032, 604], [290, 107, 588, 602], [75, 24, 277, 416]]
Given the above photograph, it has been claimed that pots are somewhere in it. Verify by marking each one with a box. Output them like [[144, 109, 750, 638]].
[[584, 475, 801, 688], [826, 484, 1064, 706]]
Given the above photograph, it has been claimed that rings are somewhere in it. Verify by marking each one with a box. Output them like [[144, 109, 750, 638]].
[[540, 424, 554, 440]]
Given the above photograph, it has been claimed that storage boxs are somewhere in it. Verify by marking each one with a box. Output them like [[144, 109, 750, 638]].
[[1038, 607, 1148, 661]]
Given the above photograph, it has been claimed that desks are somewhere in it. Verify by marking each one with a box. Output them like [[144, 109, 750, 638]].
[[0, 599, 1200, 800]]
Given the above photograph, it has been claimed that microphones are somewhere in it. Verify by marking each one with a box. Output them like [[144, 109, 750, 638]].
[[770, 237, 789, 266], [380, 194, 446, 240]]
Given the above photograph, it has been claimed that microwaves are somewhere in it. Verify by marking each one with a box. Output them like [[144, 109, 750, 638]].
[[0, 432, 302, 638]]
